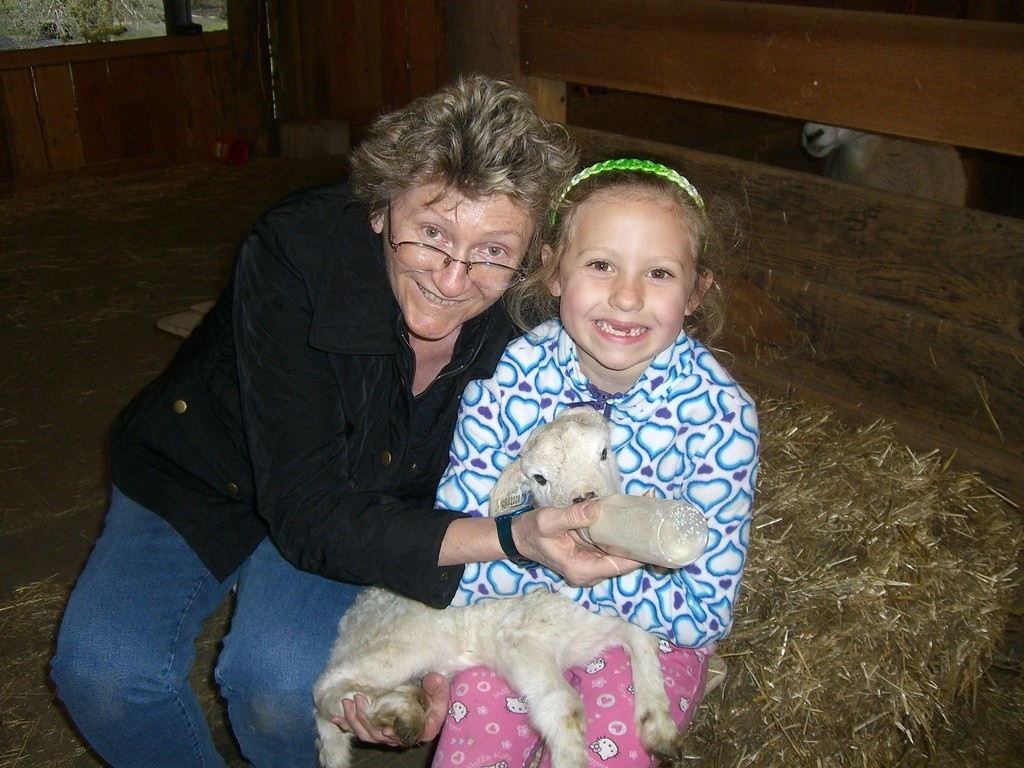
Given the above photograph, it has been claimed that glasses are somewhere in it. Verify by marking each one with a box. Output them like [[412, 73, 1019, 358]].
[[387, 199, 527, 290]]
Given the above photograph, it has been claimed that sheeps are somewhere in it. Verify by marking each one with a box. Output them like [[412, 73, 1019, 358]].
[[802, 121, 966, 208], [312, 403, 685, 768]]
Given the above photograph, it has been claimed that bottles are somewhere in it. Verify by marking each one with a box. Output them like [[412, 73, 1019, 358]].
[[576, 492, 711, 570]]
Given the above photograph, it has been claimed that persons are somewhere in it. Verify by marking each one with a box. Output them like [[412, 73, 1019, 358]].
[[432, 141, 762, 768], [46, 70, 665, 768]]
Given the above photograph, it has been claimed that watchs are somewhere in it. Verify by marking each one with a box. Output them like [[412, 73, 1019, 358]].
[[492, 503, 544, 573]]
